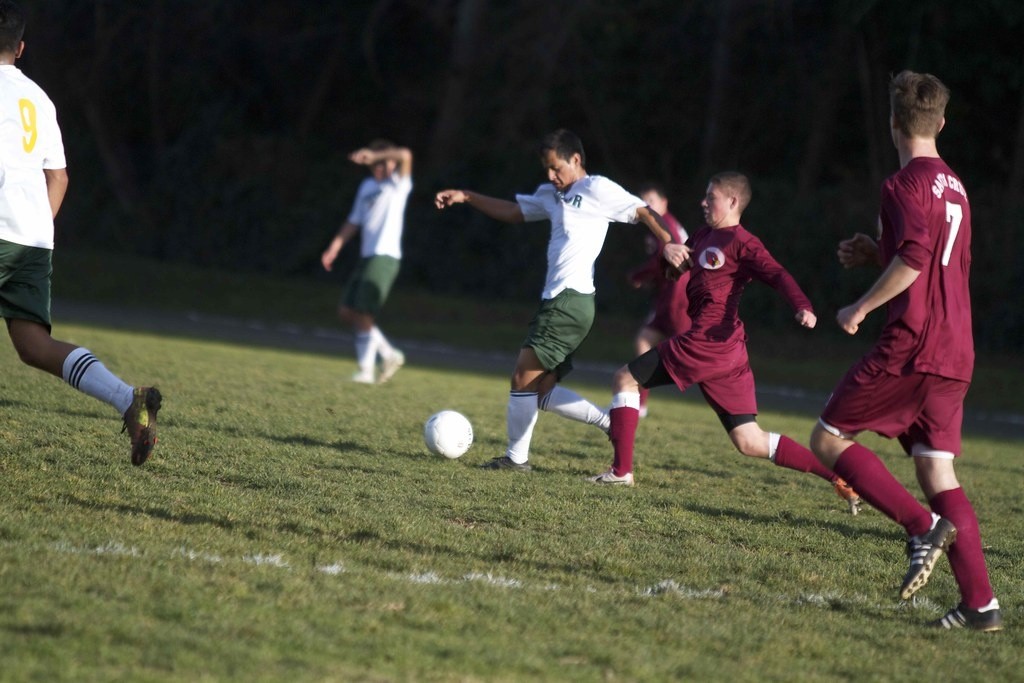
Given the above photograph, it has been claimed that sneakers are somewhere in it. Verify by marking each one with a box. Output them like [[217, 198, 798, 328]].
[[836, 477, 860, 518], [378, 353, 405, 384], [898, 515, 957, 600], [353, 372, 375, 384], [489, 456, 531, 472], [929, 603, 1005, 632], [587, 470, 635, 488], [123, 387, 162, 467]]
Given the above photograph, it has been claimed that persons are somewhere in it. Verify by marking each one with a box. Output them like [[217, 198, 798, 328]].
[[1, 0, 163, 469], [633, 184, 690, 421], [321, 135, 415, 385], [433, 127, 695, 473], [810, 69, 1006, 638], [583, 167, 866, 518]]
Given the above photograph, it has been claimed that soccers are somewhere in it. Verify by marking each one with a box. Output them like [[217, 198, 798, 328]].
[[424, 409, 474, 459]]
